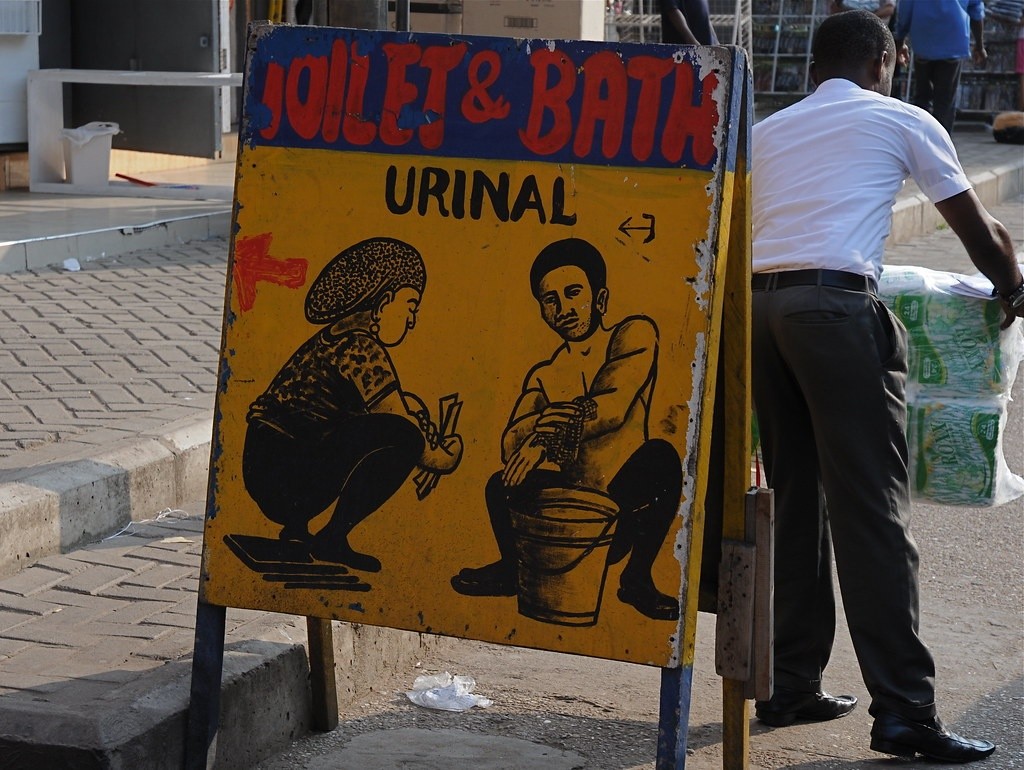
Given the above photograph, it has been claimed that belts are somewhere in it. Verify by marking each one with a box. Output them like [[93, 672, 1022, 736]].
[[751, 268, 877, 292]]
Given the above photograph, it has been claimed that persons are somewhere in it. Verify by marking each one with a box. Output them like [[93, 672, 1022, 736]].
[[752, 9, 1024, 763], [1017, 9, 1024, 113], [830, 0, 895, 28], [661, 0, 720, 44], [896, 0, 988, 136]]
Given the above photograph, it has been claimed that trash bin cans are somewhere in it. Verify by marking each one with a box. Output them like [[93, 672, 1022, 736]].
[[62, 129, 112, 186]]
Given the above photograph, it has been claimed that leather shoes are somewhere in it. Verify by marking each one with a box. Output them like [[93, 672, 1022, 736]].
[[758, 694, 858, 727], [870, 714, 996, 762]]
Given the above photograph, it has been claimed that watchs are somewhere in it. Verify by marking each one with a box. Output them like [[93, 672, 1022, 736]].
[[992, 275, 1024, 308]]
